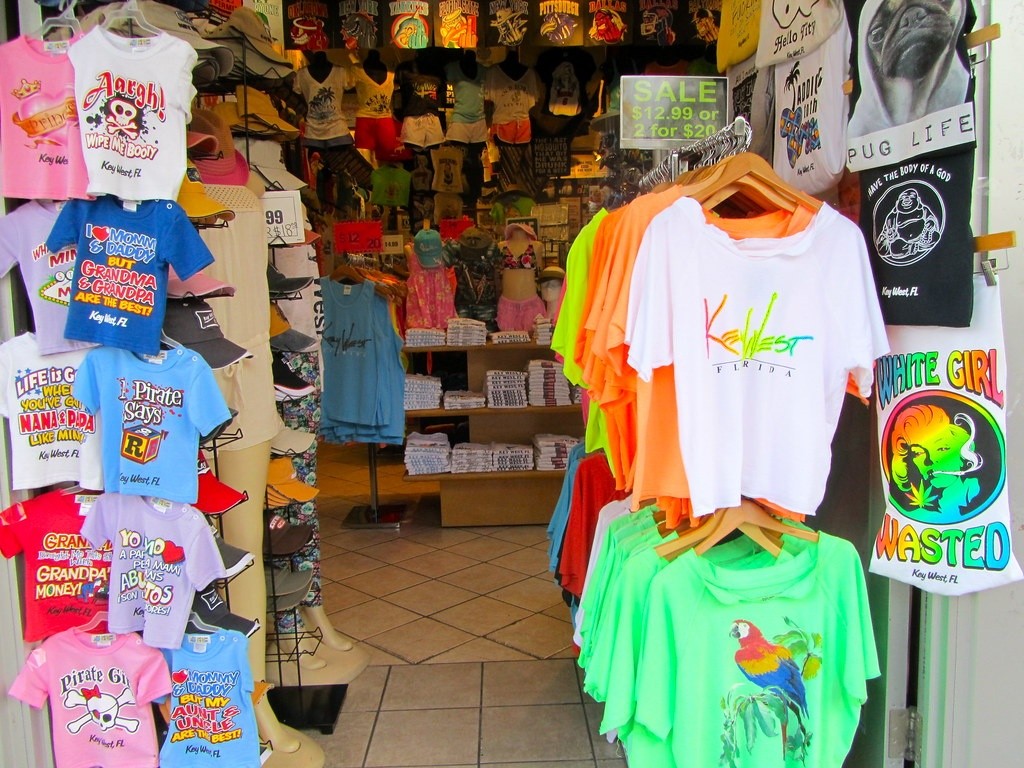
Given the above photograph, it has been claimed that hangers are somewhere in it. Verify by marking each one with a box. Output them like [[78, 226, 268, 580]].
[[71, 611, 109, 634], [92, 0, 163, 37], [637, 121, 826, 213], [161, 325, 185, 352], [328, 253, 405, 306], [25, 0, 85, 42], [629, 498, 820, 559], [62, 481, 103, 496], [186, 611, 229, 635]]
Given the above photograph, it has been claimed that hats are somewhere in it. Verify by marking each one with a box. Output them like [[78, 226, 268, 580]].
[[504, 224, 538, 240], [200, 525, 255, 578], [267, 581, 312, 613], [262, 515, 317, 553], [74, 1, 322, 514], [185, 581, 260, 637], [265, 566, 312, 596], [462, 227, 492, 249], [540, 266, 564, 280], [414, 229, 443, 268]]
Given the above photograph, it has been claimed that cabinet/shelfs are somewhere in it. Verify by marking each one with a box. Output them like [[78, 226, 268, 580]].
[[402, 339, 587, 481]]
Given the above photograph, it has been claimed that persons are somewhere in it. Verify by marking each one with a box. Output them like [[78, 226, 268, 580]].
[[484, 49, 544, 146], [404, 229, 460, 330], [536, 45, 596, 122], [393, 56, 447, 148], [293, 51, 355, 147], [269, 350, 353, 670], [346, 49, 405, 161], [492, 224, 548, 338], [539, 267, 566, 317], [443, 50, 489, 143]]
